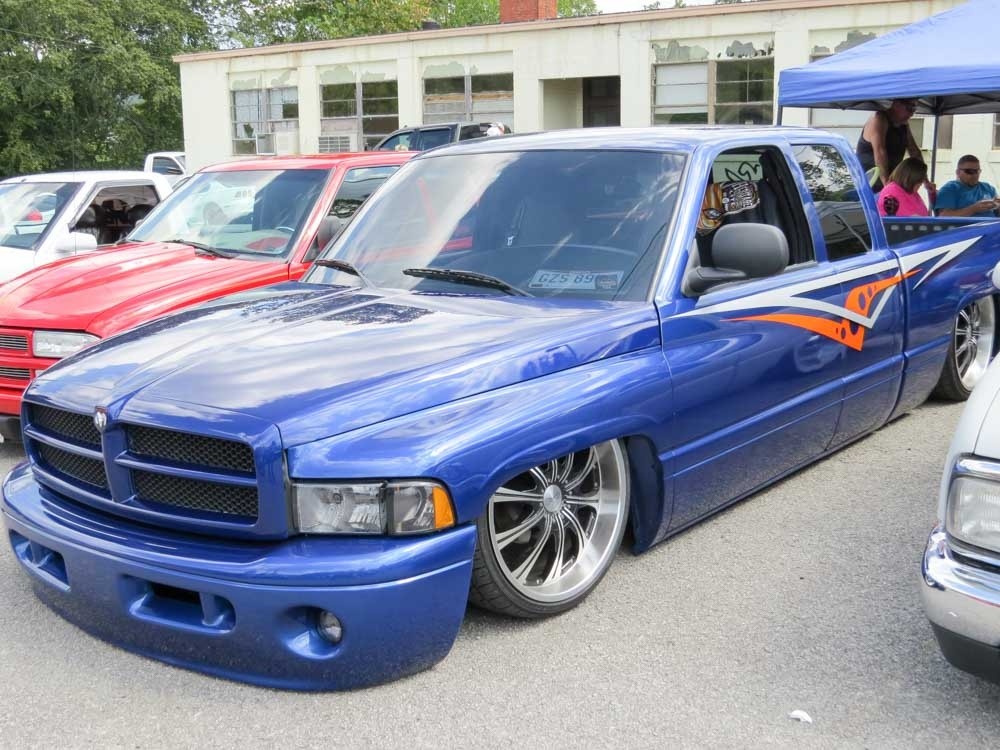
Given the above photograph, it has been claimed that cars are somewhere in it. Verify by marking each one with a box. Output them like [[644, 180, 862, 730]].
[[921, 349, 1000, 687], [169, 176, 255, 220]]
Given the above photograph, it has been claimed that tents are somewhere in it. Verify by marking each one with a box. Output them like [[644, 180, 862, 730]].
[[778, 1, 1000, 126]]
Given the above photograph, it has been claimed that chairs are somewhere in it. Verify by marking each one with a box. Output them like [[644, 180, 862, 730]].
[[72, 204, 153, 246]]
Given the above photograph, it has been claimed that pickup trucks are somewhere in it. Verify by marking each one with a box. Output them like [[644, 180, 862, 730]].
[[145, 150, 185, 180], [2, 126, 1000, 694]]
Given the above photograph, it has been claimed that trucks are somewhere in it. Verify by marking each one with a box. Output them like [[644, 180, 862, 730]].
[[0, 170, 184, 286]]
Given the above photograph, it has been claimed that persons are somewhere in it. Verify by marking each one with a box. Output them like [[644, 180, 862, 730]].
[[854, 98, 937, 191], [876, 155, 930, 218], [934, 154, 1000, 216]]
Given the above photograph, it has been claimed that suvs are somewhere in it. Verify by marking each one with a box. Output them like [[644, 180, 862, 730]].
[[2, 148, 475, 429]]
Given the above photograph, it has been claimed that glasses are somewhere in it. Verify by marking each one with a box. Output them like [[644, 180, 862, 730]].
[[899, 102, 917, 113], [959, 168, 982, 174]]
